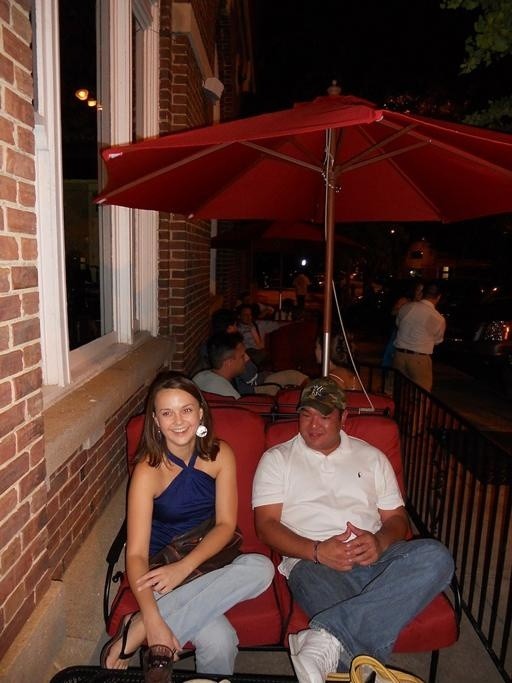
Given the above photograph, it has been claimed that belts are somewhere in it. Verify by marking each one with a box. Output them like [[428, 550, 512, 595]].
[[394, 346, 432, 356]]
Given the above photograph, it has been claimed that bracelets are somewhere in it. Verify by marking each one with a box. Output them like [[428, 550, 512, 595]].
[[313, 541, 320, 564]]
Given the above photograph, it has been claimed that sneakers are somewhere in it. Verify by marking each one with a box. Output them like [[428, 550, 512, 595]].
[[288, 628, 343, 683]]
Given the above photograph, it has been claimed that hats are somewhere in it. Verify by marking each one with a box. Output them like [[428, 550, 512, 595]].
[[295, 376, 346, 416]]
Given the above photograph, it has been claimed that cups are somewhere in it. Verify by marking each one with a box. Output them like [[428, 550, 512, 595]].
[[141, 643, 175, 683]]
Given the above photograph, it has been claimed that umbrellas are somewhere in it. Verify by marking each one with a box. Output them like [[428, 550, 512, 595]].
[[91, 79, 512, 378], [210, 221, 365, 310]]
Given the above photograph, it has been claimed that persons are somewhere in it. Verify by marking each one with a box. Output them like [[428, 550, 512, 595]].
[[100, 370, 275, 675], [251, 373, 456, 683], [192, 268, 447, 437]]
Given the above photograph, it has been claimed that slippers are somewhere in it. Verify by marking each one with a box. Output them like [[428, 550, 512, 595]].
[[100, 611, 142, 670]]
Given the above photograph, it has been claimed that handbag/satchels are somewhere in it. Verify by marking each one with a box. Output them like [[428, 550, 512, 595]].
[[150, 517, 243, 592]]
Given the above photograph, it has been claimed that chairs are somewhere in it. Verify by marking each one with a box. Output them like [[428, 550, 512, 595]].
[[103, 382, 461, 683]]
